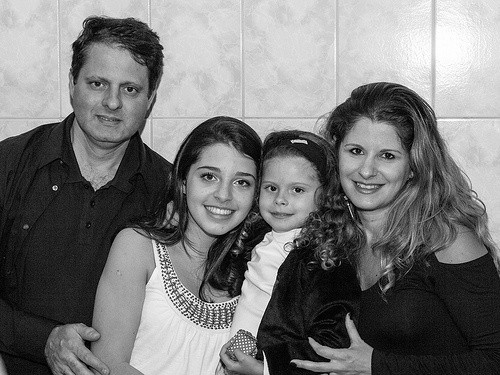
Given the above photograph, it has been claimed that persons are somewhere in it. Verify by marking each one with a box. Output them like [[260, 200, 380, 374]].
[[216, 131, 338, 374], [86, 115, 271, 375], [257, 81, 500, 374], [0, 15, 176, 375]]
